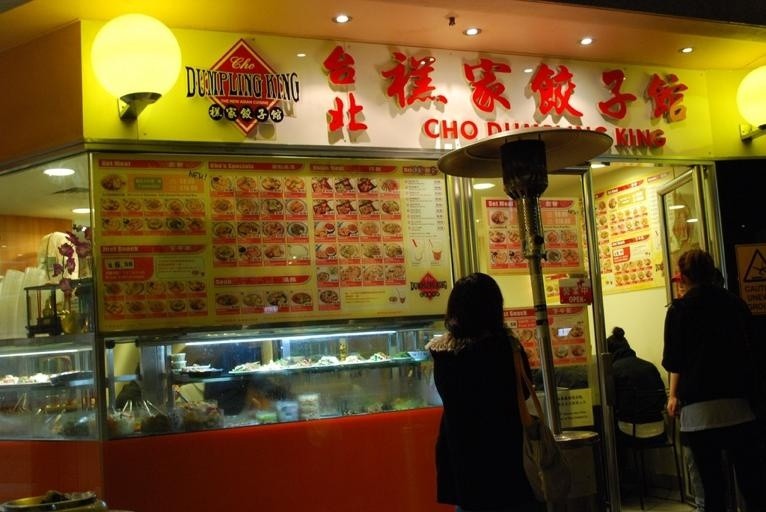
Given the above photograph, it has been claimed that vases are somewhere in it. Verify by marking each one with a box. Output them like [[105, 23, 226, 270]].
[[56, 292, 84, 335]]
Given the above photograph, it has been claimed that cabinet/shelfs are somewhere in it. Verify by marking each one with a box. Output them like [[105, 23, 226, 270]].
[[0, 318, 456, 511]]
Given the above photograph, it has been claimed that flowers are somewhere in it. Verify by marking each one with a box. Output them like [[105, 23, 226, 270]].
[[51, 225, 94, 311]]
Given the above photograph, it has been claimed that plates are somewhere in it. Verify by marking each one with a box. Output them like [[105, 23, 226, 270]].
[[212, 176, 308, 238]]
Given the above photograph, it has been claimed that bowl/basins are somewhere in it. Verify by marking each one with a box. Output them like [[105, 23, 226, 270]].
[[408, 351, 431, 361]]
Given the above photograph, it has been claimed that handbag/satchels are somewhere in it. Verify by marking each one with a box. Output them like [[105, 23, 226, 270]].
[[520, 413, 573, 512]]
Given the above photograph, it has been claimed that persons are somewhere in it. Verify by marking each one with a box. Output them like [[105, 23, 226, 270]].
[[425, 270, 533, 512], [662, 247, 766, 512], [605, 327, 674, 465]]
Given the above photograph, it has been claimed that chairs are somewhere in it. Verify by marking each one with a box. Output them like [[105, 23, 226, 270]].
[[617, 384, 689, 510]]
